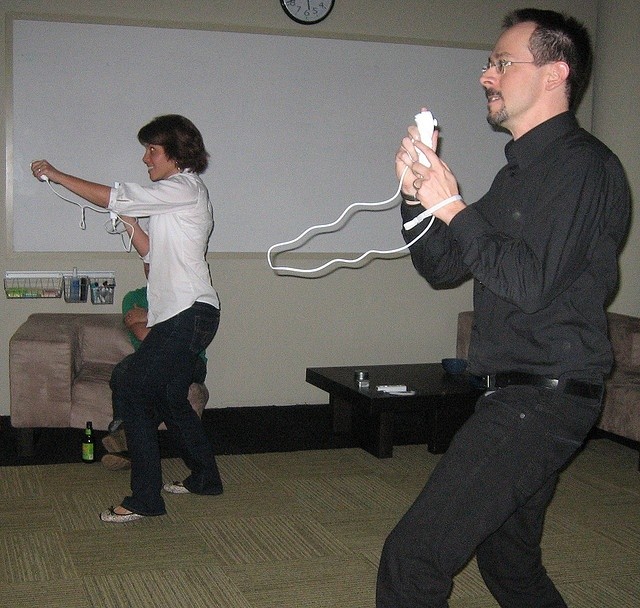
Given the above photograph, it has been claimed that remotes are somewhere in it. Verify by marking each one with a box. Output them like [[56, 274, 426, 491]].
[[376, 384, 407, 392]]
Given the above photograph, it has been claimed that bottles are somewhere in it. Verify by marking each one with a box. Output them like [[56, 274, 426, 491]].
[[81, 420, 99, 463]]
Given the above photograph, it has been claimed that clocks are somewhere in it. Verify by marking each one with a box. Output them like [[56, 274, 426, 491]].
[[279, 0, 333, 25]]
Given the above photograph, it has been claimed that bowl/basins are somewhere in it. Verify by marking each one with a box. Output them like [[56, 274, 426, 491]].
[[442, 356, 473, 386]]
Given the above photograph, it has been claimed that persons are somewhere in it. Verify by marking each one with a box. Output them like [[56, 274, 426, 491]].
[[375, 8, 631, 608], [101, 263, 209, 472], [30, 114, 221, 523]]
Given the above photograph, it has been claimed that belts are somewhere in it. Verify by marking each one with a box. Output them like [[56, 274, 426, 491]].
[[473, 370, 603, 400]]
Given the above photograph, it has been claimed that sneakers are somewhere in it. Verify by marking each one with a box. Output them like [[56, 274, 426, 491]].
[[99, 506, 146, 522], [164, 479, 193, 494]]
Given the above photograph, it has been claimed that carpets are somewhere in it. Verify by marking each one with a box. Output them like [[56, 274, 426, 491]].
[[1, 443, 639, 608]]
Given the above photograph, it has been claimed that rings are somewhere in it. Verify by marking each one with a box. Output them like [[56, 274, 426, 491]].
[[128, 314, 132, 317], [38, 168, 41, 173]]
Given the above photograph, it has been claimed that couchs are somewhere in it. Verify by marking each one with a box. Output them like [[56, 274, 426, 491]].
[[10, 313, 207, 434], [456, 310, 640, 442]]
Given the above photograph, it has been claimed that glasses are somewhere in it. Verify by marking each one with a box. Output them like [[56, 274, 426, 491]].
[[482, 58, 552, 73]]
